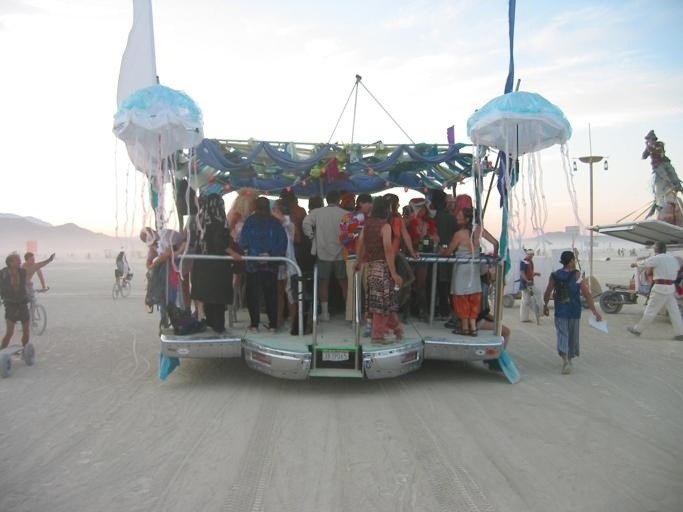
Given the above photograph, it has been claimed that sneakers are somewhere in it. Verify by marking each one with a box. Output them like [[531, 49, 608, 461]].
[[627, 327, 641, 336], [248, 320, 312, 336], [361, 328, 404, 347], [560, 363, 575, 374], [318, 312, 330, 322], [207, 326, 235, 336], [418, 311, 478, 336], [674, 335, 683, 342]]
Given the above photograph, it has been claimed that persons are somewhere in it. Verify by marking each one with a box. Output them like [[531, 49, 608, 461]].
[[114, 249, 131, 287], [543, 250, 603, 375], [139, 182, 499, 344], [518, 246, 543, 324], [642, 127, 682, 224], [20, 251, 47, 313], [625, 239, 682, 342], [1, 251, 57, 349]]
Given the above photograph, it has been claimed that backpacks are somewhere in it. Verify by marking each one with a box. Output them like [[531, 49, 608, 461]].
[[551, 269, 579, 304], [166, 303, 201, 335], [145, 267, 163, 313]]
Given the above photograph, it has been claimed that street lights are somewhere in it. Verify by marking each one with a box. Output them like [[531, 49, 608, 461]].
[[572, 154, 610, 303]]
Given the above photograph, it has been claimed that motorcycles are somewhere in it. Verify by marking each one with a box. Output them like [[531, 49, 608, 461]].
[[599, 263, 637, 313]]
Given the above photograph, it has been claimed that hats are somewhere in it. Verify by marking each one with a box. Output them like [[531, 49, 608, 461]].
[[646, 130, 658, 140], [408, 198, 425, 206]]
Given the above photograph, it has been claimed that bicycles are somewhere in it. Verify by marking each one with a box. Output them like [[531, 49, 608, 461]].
[[110, 269, 133, 302], [14, 287, 52, 339]]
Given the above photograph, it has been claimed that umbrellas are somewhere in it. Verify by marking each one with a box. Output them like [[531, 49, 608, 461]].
[[468, 87, 568, 175], [112, 85, 203, 157]]
